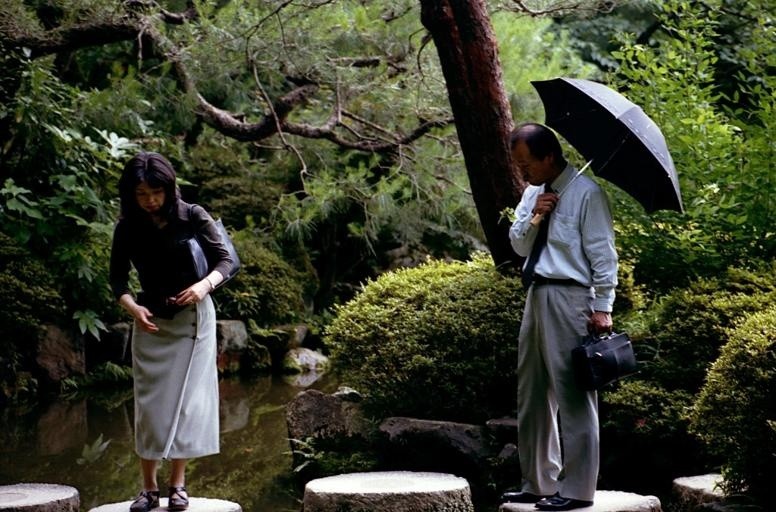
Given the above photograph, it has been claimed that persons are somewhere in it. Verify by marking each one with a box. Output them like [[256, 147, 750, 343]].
[[505, 122, 618, 510], [107, 147, 241, 512]]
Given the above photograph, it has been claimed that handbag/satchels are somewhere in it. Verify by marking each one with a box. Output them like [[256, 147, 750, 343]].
[[178, 216, 241, 292], [569, 332, 637, 392]]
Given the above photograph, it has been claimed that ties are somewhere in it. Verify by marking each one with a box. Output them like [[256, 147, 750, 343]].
[[521, 185, 554, 293]]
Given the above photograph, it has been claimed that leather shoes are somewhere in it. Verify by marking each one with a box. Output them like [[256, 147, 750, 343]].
[[169, 486, 189, 509], [503, 492, 593, 510], [130, 490, 160, 512]]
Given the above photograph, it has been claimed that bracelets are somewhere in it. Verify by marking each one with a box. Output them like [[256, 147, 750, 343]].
[[207, 277, 216, 293]]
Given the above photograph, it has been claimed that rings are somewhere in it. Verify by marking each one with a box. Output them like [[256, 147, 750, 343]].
[[191, 302, 194, 304]]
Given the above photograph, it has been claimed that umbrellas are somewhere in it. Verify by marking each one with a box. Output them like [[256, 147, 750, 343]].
[[529, 72, 684, 230]]
